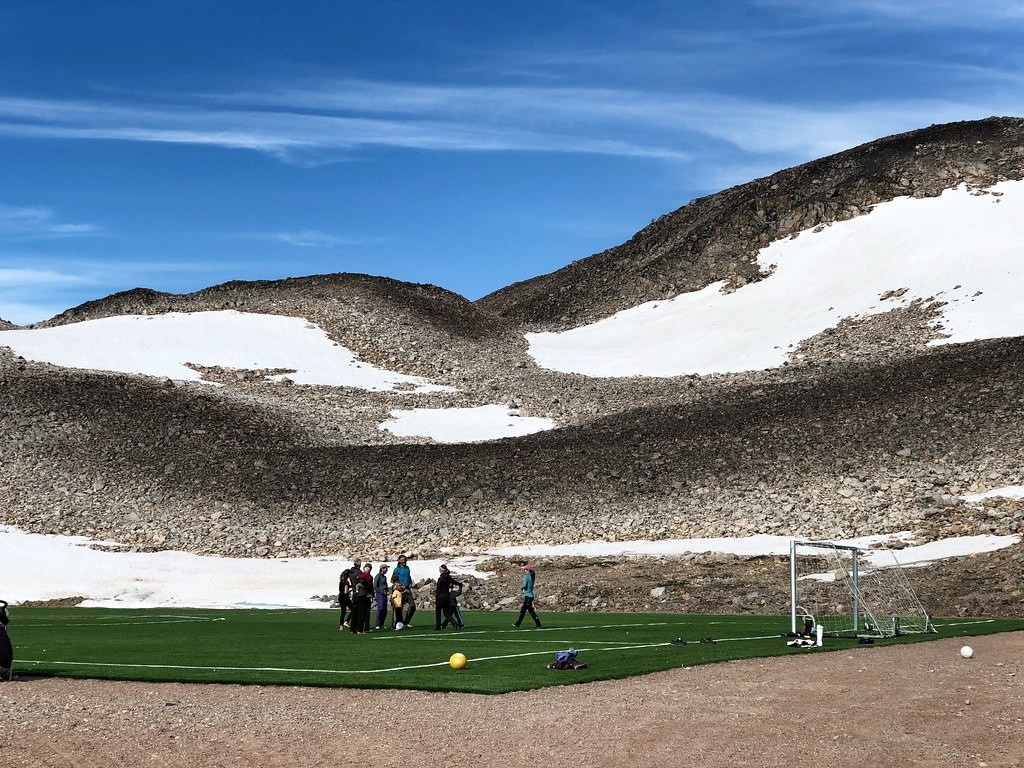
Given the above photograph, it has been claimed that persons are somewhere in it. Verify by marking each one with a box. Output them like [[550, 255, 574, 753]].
[[512, 565, 543, 629], [339, 555, 464, 635]]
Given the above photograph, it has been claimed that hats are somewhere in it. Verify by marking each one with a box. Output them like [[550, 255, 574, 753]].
[[353, 558, 361, 565], [363, 563, 372, 569], [380, 564, 390, 568], [521, 565, 532, 570]]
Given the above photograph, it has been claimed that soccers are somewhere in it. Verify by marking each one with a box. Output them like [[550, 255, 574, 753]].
[[448, 651, 468, 672], [960, 645, 974, 660]]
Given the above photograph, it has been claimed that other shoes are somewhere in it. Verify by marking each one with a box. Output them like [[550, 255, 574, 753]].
[[700, 637, 716, 644], [534, 625, 542, 628], [671, 637, 687, 646], [384, 627, 387, 629], [370, 629, 372, 631], [343, 621, 350, 627], [440, 625, 446, 629], [454, 623, 458, 630], [357, 631, 366, 634], [376, 625, 380, 629], [391, 627, 394, 631], [460, 624, 464, 628], [403, 624, 412, 627], [339, 625, 344, 630], [433, 627, 441, 630], [512, 623, 519, 629]]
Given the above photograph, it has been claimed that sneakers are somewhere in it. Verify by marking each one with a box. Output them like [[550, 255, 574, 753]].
[[568, 655, 587, 670], [547, 660, 570, 670]]
